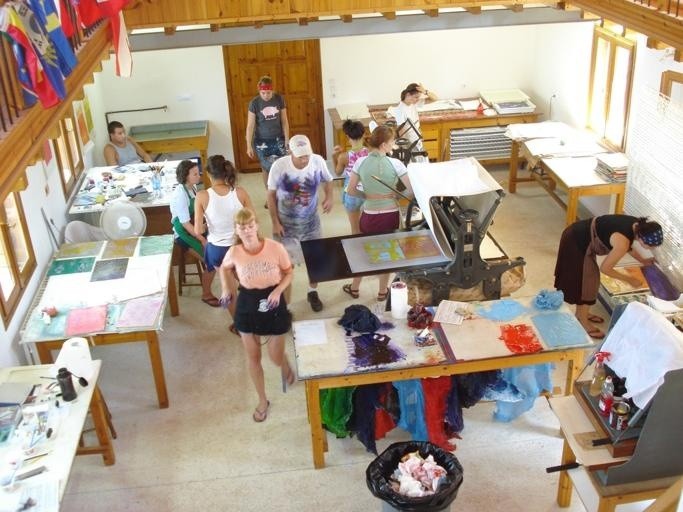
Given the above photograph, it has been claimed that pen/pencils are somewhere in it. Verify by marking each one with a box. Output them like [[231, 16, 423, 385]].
[[45, 382, 56, 389], [24, 453, 48, 461], [41, 393, 62, 402], [49, 384, 60, 390], [148, 165, 164, 174], [40, 376, 57, 380]]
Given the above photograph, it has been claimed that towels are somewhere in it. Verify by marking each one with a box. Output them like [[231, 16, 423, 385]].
[[599, 300, 682, 412]]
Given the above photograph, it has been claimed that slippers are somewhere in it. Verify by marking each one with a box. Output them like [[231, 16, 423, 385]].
[[282, 362, 294, 385], [201, 297, 221, 306], [587, 328, 605, 339], [253, 400, 269, 422], [587, 315, 604, 323]]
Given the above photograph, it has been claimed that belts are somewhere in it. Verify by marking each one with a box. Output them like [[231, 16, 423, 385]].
[[363, 207, 399, 214]]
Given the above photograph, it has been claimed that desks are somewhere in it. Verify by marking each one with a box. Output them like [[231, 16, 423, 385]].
[[554, 421, 681, 512], [291, 291, 597, 471], [66, 157, 198, 264], [0, 359, 116, 512], [127, 120, 209, 190], [507, 122, 626, 229], [443, 93, 544, 168], [326, 101, 442, 191], [26, 234, 180, 410]]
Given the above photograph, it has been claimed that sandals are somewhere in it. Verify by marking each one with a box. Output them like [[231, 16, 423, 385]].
[[230, 323, 241, 337], [377, 287, 389, 301], [343, 284, 359, 298]]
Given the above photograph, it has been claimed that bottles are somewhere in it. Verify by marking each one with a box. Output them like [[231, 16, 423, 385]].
[[56, 366, 88, 402], [597, 375, 615, 417]]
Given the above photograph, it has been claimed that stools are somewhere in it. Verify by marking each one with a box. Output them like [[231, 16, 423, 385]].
[[176, 244, 207, 297], [79, 382, 116, 448]]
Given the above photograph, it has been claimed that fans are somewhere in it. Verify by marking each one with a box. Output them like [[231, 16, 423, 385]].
[[96, 199, 146, 241]]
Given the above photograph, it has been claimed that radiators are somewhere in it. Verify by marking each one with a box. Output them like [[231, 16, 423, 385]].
[[19, 249, 57, 366]]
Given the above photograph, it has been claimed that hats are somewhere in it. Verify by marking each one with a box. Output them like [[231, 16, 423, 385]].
[[288, 134, 312, 158]]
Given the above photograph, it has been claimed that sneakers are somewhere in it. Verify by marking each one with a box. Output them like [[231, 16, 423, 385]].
[[308, 291, 322, 311]]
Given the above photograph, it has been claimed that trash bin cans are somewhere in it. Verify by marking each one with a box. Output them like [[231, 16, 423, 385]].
[[366, 441, 464, 512]]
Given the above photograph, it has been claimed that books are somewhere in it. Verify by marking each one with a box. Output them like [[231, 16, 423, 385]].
[[491, 98, 536, 115], [596, 152, 627, 182]]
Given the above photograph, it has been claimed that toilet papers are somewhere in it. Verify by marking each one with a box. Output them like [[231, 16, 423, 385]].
[[387, 279, 412, 322], [46, 330, 98, 389]]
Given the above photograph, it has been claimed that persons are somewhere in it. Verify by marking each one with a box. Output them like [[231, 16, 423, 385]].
[[343, 125, 414, 301], [384, 81, 438, 163], [331, 121, 373, 234], [219, 207, 293, 423], [103, 121, 153, 167], [246, 75, 290, 210], [267, 135, 334, 312], [168, 160, 223, 307], [553, 214, 663, 339], [194, 155, 260, 335]]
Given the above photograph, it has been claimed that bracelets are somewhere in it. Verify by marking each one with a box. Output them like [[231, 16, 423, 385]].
[[425, 90, 429, 95]]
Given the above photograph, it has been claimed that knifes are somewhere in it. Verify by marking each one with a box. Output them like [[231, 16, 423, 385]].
[[592, 426, 641, 448]]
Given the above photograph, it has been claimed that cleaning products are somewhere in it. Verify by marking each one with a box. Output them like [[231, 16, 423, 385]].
[[474, 96, 488, 117], [595, 372, 617, 420], [585, 349, 613, 401]]
